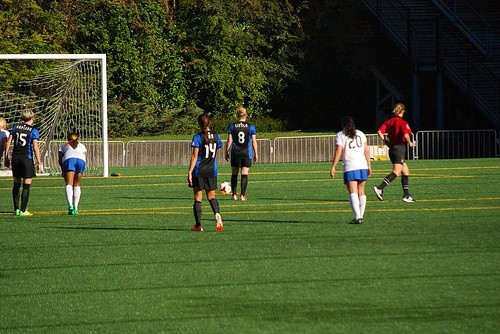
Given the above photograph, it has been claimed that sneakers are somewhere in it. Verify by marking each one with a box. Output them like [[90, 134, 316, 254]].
[[349, 218, 362, 224], [15, 209, 20, 215], [402, 194, 416, 203], [68, 206, 74, 215], [240, 194, 247, 200], [373, 186, 384, 201], [19, 209, 34, 216], [361, 219, 365, 223], [214, 212, 224, 232], [232, 193, 238, 200], [191, 225, 203, 231], [72, 209, 78, 215]]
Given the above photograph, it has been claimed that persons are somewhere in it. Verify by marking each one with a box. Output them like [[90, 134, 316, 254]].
[[188, 114, 224, 232], [330, 116, 372, 224], [0, 117, 10, 169], [225, 107, 258, 202], [4, 109, 43, 217], [58, 132, 87, 215], [373, 103, 417, 202]]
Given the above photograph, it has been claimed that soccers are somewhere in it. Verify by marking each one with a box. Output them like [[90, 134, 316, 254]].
[[220, 181, 231, 194]]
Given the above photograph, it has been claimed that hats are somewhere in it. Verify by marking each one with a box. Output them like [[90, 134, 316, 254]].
[[21, 109, 35, 119]]
